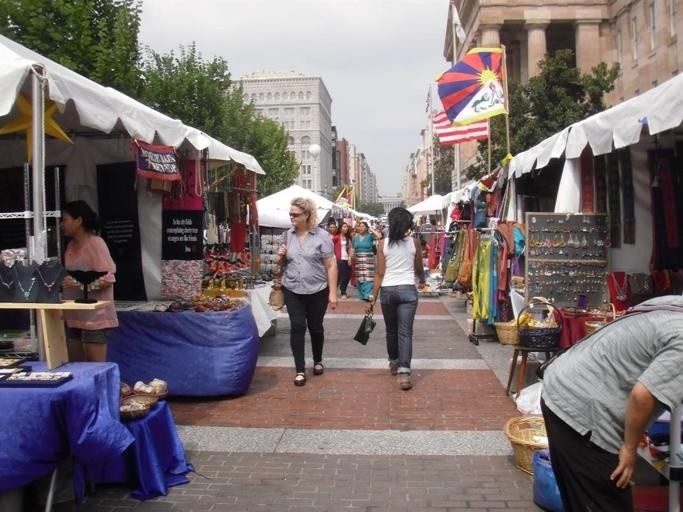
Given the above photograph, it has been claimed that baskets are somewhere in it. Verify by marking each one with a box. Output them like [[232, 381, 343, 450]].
[[119, 381, 169, 421], [495, 322, 520, 345], [585, 303, 615, 336], [504, 416, 549, 476], [517, 303, 563, 349]]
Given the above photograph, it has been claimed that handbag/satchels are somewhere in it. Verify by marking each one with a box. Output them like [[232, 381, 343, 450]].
[[353, 315, 376, 345], [269, 282, 285, 311], [474, 209, 487, 227], [443, 229, 480, 288]]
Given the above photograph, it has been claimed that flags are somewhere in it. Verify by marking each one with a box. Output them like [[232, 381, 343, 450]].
[[434, 47, 509, 126], [432, 107, 491, 146]]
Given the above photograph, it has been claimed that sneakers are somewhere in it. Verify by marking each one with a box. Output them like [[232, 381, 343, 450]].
[[398, 374, 411, 389], [389, 362, 399, 376]]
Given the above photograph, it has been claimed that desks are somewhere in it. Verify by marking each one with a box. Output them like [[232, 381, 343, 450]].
[[0, 359, 137, 511], [120, 399, 194, 502]]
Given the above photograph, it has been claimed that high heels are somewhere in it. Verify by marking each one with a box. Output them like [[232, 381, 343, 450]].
[[294, 372, 306, 386], [314, 362, 324, 375]]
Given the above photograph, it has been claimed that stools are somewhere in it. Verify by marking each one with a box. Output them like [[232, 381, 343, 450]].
[[506, 344, 561, 408]]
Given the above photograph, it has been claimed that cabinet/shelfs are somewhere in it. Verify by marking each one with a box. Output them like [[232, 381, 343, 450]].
[[637, 403, 682, 512], [0, 299, 112, 370]]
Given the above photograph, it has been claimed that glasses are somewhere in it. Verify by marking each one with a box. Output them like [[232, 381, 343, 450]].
[[289, 212, 302, 217]]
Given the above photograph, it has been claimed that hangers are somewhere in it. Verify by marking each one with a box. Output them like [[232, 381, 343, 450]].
[[476, 228, 499, 242]]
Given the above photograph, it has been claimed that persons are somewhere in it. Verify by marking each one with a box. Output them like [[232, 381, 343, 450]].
[[538, 295, 683, 512], [275, 196, 339, 386], [363, 207, 425, 390], [58, 200, 120, 362], [326, 217, 430, 301]]
